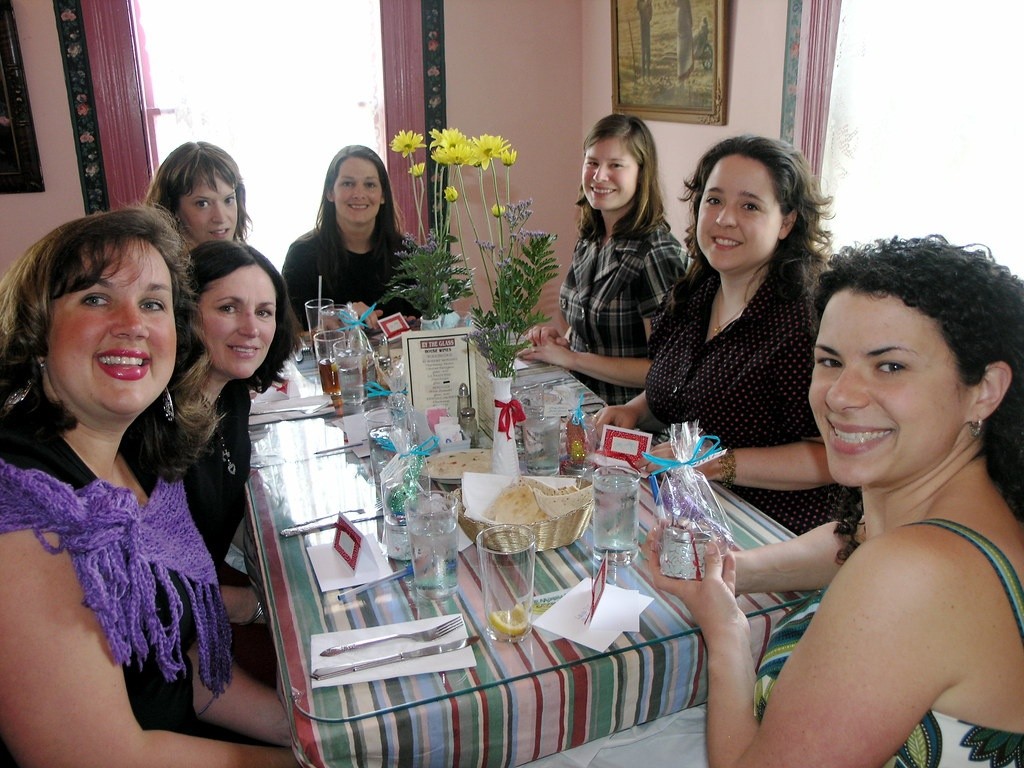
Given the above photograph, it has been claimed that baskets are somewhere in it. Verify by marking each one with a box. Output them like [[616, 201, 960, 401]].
[[447, 474, 595, 552]]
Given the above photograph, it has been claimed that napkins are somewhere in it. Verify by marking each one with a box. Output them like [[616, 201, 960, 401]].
[[249, 395, 335, 424], [310, 613, 477, 688]]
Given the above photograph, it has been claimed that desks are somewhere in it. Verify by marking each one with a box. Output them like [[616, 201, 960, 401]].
[[243, 310, 836, 768]]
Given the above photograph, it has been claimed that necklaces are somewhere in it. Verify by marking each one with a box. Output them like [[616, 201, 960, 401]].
[[714, 291, 744, 334]]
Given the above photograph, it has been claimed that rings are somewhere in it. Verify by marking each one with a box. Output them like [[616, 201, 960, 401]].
[[594, 415, 598, 420], [532, 349, 536, 353]]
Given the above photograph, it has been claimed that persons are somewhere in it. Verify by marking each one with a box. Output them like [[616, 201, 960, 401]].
[[591, 134, 853, 536], [517, 114, 686, 409], [282, 145, 428, 331], [0, 207, 300, 768], [157, 239, 302, 691], [146, 141, 253, 251], [645, 233, 1024, 768]]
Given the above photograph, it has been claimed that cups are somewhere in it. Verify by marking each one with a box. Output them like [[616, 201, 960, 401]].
[[304, 299, 335, 358], [333, 339, 367, 403], [404, 490, 459, 600], [476, 525, 536, 643], [363, 406, 408, 486], [313, 330, 346, 396], [510, 379, 563, 477], [592, 465, 640, 567], [452, 310, 470, 328], [319, 304, 349, 359]]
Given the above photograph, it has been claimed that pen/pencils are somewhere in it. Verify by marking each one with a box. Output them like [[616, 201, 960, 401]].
[[362, 397, 369, 404], [314, 442, 363, 455], [649, 475, 667, 520], [336, 563, 413, 601]]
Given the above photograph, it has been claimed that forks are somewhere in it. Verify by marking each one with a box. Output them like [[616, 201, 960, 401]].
[[320, 616, 464, 657], [286, 500, 383, 529], [248, 401, 329, 415]]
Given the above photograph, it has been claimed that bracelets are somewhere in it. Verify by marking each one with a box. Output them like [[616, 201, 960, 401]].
[[238, 602, 263, 626], [718, 449, 737, 488]]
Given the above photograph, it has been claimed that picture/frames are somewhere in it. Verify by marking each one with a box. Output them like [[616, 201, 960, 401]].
[[610, 0, 727, 124], [0, 0, 46, 194]]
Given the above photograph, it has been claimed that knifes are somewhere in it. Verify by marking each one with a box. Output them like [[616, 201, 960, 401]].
[[309, 636, 481, 680], [279, 510, 383, 538]]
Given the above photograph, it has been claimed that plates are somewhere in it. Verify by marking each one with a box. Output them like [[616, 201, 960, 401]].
[[417, 449, 492, 485]]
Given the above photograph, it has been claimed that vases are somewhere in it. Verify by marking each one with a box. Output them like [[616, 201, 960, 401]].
[[419, 315, 443, 332], [487, 373, 522, 479]]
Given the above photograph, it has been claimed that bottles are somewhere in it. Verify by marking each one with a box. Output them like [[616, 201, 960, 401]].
[[459, 408, 479, 448], [376, 333, 392, 389], [456, 383, 471, 424]]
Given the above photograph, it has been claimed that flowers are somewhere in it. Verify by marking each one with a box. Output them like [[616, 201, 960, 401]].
[[379, 128, 559, 376]]
